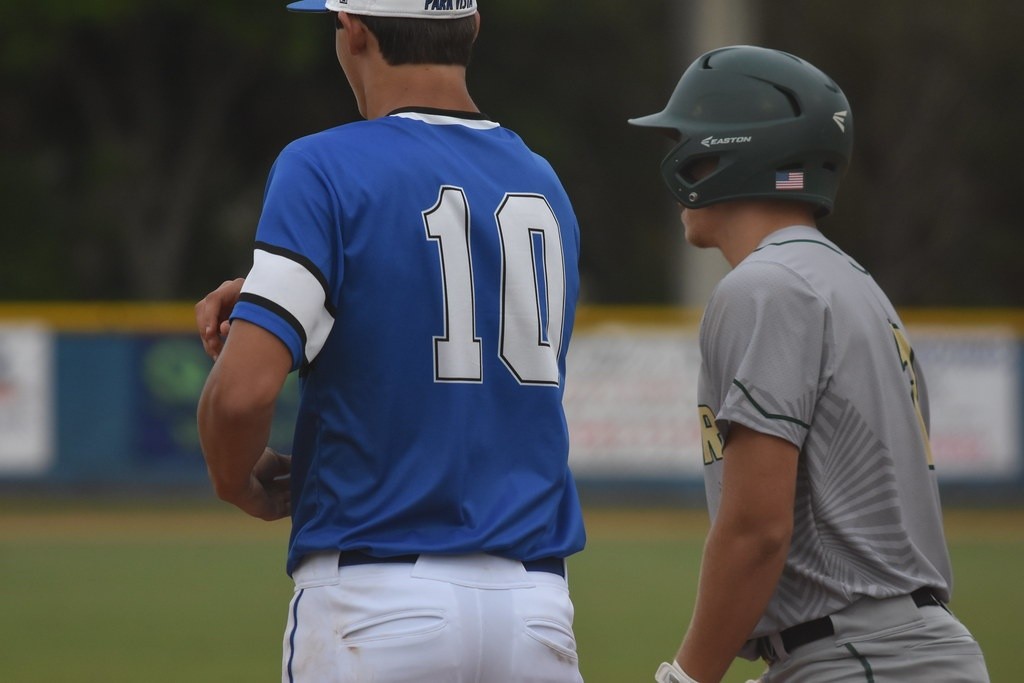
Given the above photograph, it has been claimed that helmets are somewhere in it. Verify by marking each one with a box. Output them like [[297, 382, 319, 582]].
[[627, 45, 856, 222]]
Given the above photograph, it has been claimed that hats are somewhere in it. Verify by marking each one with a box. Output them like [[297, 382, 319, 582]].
[[285, 0, 479, 20]]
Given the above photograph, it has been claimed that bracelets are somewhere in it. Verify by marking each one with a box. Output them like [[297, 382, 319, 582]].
[[653, 658, 700, 683]]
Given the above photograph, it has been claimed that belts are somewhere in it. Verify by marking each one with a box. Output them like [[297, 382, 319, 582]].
[[757, 585, 943, 665], [338, 549, 565, 577]]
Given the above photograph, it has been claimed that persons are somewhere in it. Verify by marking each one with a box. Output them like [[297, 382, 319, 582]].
[[627, 43, 992, 683], [193, 0, 587, 683]]
